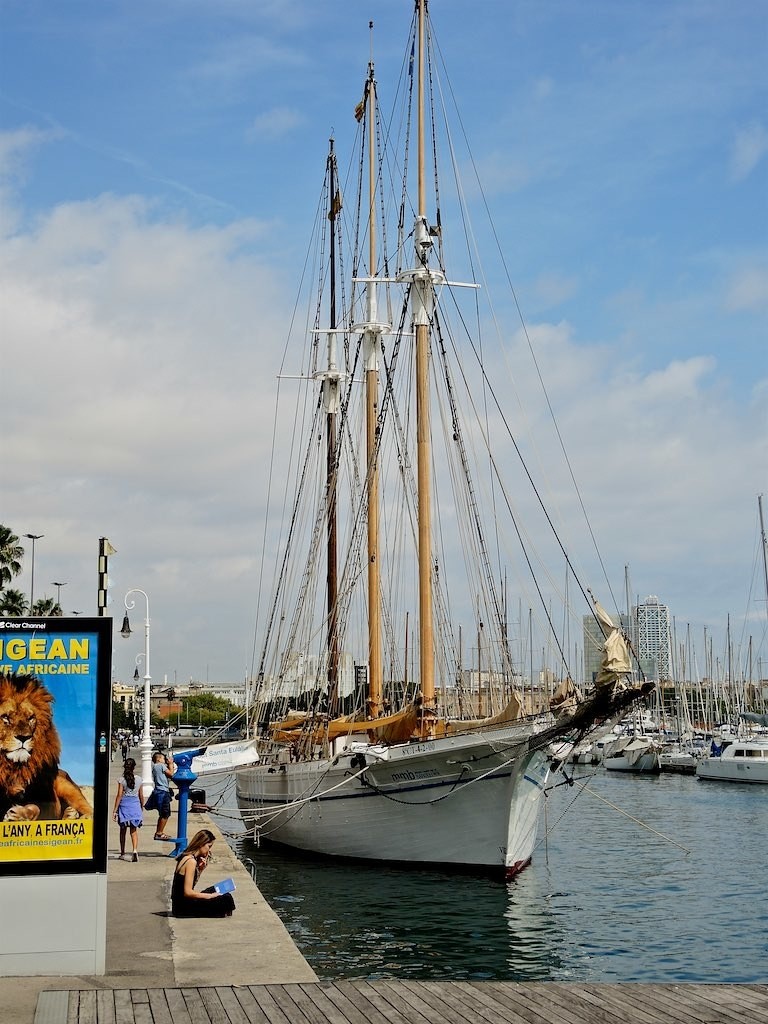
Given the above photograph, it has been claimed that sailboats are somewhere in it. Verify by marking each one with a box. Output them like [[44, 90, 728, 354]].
[[549, 490, 768, 788], [230, 1, 661, 886]]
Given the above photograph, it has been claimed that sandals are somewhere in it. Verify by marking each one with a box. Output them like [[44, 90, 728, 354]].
[[154, 832, 171, 840]]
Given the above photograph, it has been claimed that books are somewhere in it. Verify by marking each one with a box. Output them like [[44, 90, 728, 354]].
[[214, 878, 236, 895]]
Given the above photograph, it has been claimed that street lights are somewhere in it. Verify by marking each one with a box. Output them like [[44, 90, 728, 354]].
[[22, 534, 45, 618], [117, 589, 156, 807], [53, 581, 68, 619]]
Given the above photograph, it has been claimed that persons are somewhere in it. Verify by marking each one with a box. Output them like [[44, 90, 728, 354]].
[[152, 752, 174, 840], [171, 830, 236, 918], [112, 758, 144, 862], [111, 732, 139, 762]]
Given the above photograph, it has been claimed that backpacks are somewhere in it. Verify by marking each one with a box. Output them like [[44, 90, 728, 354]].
[[122, 738, 128, 747]]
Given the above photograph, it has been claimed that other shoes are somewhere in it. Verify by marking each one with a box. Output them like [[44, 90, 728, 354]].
[[132, 851, 139, 862], [119, 855, 126, 860]]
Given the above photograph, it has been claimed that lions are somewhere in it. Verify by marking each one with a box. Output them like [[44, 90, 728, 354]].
[[0, 671, 94, 821]]
